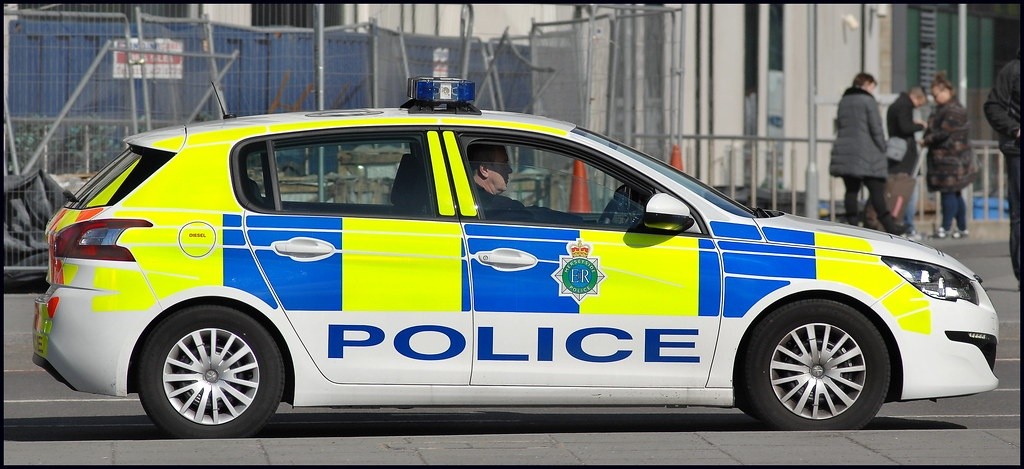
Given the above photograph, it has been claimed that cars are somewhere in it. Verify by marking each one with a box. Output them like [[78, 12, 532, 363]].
[[31, 76, 1000, 427]]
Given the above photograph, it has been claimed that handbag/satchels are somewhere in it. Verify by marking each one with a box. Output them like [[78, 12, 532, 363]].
[[886, 136, 908, 161]]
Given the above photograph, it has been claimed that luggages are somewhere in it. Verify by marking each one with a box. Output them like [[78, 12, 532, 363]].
[[862, 147, 928, 233]]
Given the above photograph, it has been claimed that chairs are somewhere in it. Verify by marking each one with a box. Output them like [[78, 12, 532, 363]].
[[389, 153, 433, 219]]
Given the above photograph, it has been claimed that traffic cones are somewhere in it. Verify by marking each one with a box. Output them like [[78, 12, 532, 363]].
[[567, 157, 591, 212], [669, 144, 684, 171]]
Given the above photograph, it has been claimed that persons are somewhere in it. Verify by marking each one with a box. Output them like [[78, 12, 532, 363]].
[[467, 144, 584, 225], [917, 73, 977, 238], [829, 73, 912, 236], [886, 84, 928, 241], [983, 33, 1020, 289]]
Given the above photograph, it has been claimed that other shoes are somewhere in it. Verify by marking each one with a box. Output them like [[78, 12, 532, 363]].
[[902, 231, 922, 242], [893, 225, 911, 236], [930, 228, 968, 239]]
